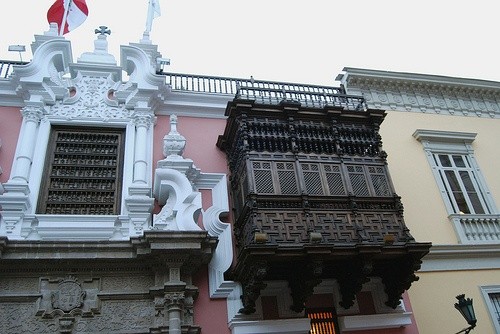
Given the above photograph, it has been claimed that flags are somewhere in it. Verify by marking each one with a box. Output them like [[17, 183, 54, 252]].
[[46, 0, 89, 37]]
